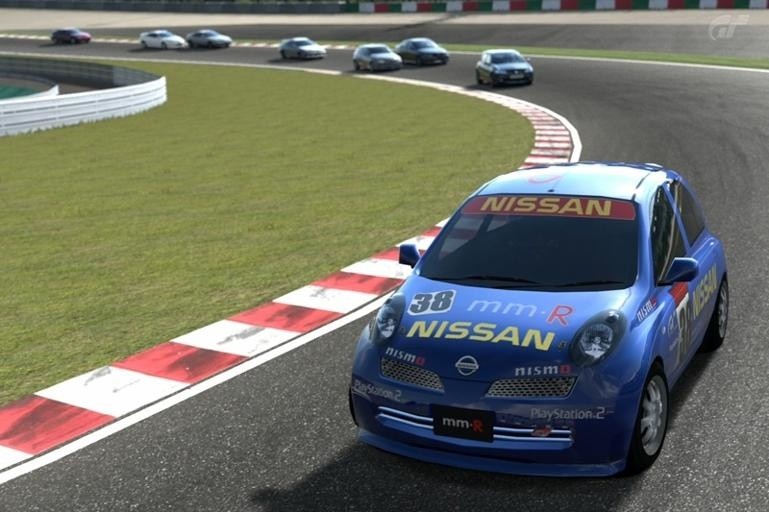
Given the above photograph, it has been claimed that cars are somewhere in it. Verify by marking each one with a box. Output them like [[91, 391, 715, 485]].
[[280, 37, 534, 88], [138, 27, 232, 50], [50, 28, 90, 43], [349, 161, 729, 477]]
